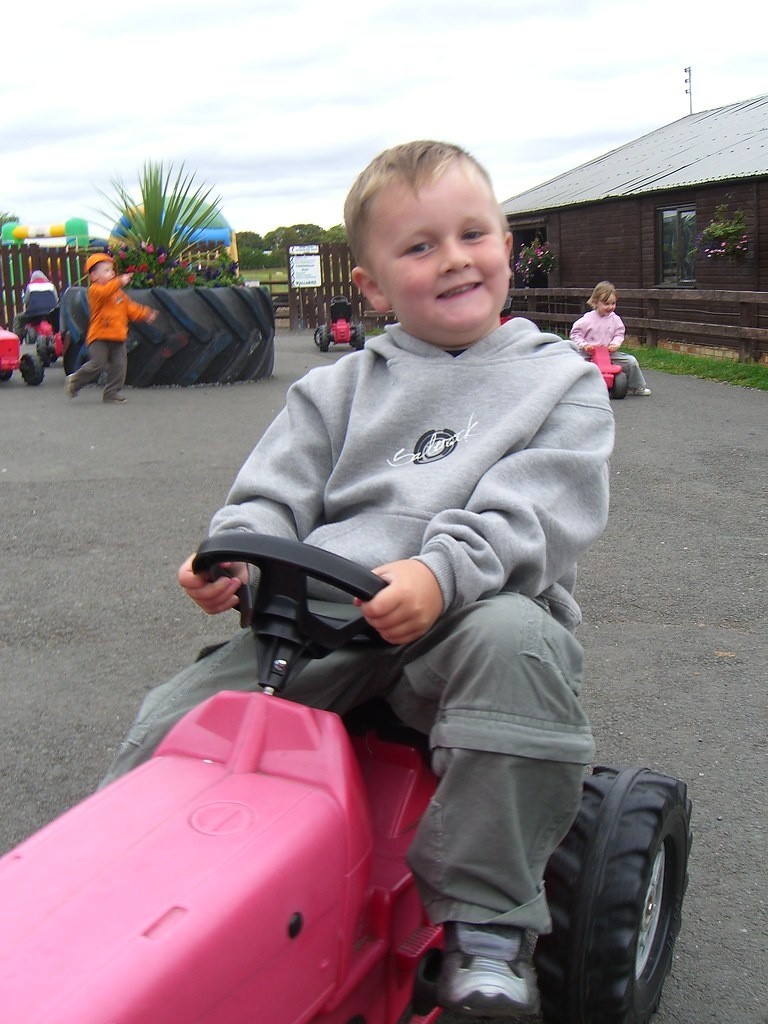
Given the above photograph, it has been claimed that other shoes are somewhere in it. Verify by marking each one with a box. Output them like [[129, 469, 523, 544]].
[[103, 394, 127, 403], [66, 373, 77, 398]]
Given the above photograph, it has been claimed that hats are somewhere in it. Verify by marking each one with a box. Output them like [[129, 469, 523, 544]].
[[84, 252, 115, 274]]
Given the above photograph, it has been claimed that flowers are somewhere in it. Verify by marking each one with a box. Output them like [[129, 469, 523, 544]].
[[692, 202, 752, 261], [514, 238, 557, 282], [97, 158, 242, 288]]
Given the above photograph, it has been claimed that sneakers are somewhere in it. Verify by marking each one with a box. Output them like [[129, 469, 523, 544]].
[[627, 387, 651, 395], [440, 919, 540, 1021]]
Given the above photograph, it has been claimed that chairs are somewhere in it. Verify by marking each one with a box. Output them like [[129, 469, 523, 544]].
[[330, 300, 352, 322], [23, 291, 59, 316], [500, 297, 513, 317]]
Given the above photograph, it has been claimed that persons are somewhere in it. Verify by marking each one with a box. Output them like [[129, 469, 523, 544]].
[[568, 282, 652, 396], [63, 254, 160, 405], [97, 138, 615, 1022], [12, 270, 59, 344]]
